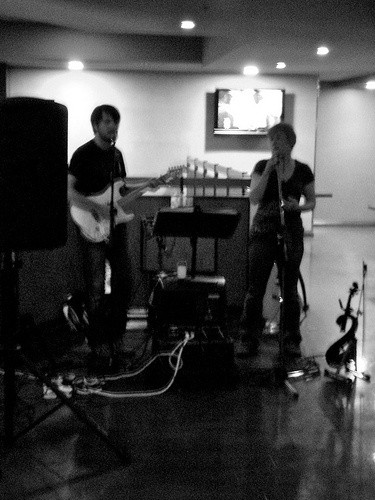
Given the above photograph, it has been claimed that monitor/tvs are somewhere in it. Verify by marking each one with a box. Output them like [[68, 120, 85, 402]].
[[213, 87, 285, 135]]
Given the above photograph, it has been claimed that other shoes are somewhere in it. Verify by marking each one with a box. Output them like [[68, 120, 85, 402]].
[[116, 341, 135, 355], [237, 341, 259, 357], [284, 340, 301, 356]]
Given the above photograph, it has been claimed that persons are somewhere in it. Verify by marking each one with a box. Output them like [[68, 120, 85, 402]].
[[65, 104, 168, 361], [234, 123, 317, 364]]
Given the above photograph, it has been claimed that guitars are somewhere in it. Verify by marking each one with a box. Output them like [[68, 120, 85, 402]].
[[68, 164, 184, 243]]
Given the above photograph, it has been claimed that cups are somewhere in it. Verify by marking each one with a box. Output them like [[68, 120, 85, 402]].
[[175, 254, 189, 280]]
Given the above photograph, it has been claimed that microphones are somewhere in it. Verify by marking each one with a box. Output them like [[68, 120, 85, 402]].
[[274, 145, 280, 165], [109, 141, 116, 173]]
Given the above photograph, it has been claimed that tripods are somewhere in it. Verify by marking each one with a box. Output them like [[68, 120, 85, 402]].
[[249, 165, 307, 397], [0, 251, 131, 467]]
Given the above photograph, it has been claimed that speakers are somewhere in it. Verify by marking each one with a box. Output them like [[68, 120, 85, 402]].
[[0, 97, 69, 251]]
